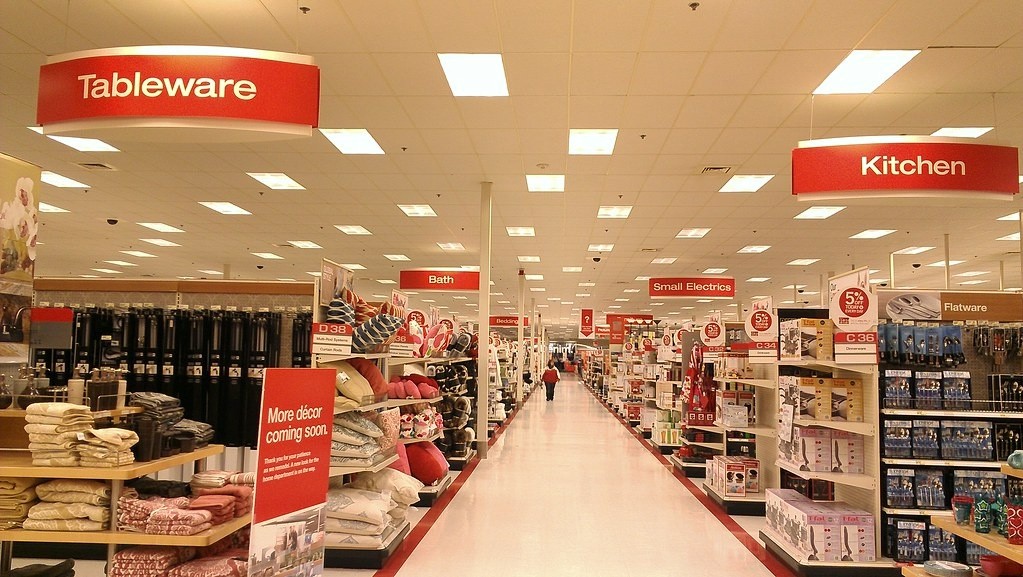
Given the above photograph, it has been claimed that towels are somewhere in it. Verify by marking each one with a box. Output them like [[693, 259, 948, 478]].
[[0, 390, 254, 577]]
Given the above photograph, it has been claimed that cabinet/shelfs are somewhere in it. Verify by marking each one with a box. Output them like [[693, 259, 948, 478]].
[[0, 406, 252, 577], [758, 362, 1008, 577], [582, 360, 780, 519], [311, 287, 410, 570], [387, 342, 550, 508]]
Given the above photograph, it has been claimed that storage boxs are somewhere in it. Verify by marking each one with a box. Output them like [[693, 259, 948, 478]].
[[610, 347, 760, 497], [765, 318, 876, 562]]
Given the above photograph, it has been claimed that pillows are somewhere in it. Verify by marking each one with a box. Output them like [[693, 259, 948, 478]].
[[391, 321, 449, 487], [316, 288, 448, 550]]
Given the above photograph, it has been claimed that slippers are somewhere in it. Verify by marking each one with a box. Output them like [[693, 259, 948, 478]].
[[446, 333, 457, 358], [451, 333, 470, 359]]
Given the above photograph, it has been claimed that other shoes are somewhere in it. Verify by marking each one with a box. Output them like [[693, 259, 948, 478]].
[[546, 396, 552, 401], [439, 398, 470, 427], [427, 364, 467, 393], [439, 429, 471, 457]]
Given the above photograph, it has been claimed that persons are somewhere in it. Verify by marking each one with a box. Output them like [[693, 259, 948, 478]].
[[540, 360, 560, 401]]
